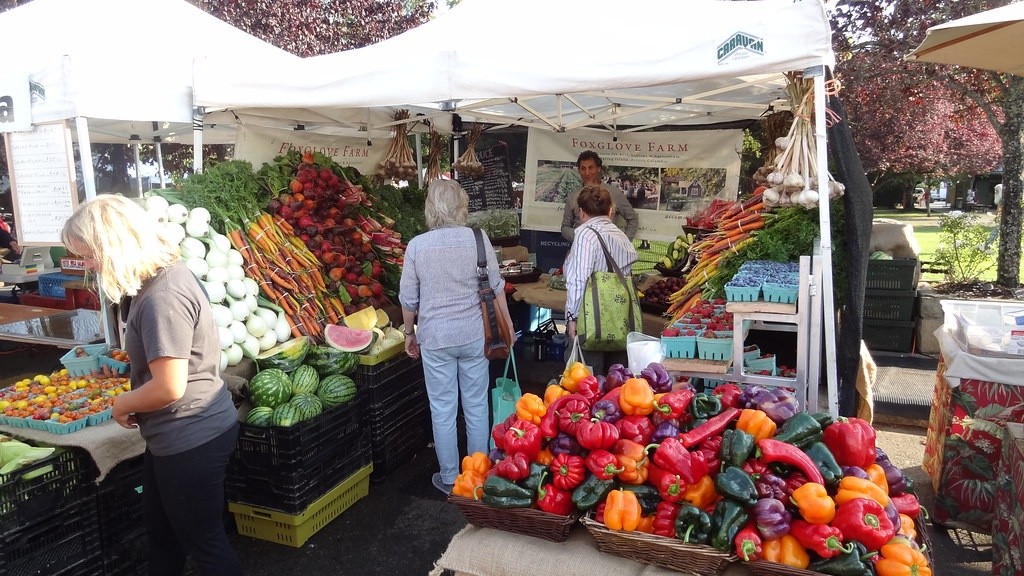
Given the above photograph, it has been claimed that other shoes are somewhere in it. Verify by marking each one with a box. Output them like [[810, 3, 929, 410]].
[[432, 472, 455, 497]]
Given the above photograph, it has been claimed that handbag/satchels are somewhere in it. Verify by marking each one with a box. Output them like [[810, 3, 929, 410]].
[[470, 225, 512, 360], [577, 227, 643, 352], [490, 347, 522, 452], [560, 333, 593, 385]]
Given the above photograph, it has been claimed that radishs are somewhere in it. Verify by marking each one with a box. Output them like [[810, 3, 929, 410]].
[[143, 195, 290, 371]]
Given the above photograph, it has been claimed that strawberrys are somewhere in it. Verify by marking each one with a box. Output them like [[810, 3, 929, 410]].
[[662, 298, 796, 392]]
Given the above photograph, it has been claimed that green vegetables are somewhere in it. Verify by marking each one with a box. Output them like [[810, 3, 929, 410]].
[[348, 166, 426, 242]]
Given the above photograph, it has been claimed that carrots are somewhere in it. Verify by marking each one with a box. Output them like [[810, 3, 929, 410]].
[[669, 195, 764, 319], [227, 212, 344, 338]]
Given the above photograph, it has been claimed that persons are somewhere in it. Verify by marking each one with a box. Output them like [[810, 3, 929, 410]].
[[923, 190, 929, 207], [968, 187, 977, 213], [560, 150, 639, 244], [0, 225, 23, 256], [59, 193, 242, 576], [561, 186, 638, 384], [398, 180, 517, 498]]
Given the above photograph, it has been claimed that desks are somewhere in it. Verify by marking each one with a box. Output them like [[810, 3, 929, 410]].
[[0, 296, 106, 356], [433, 523, 694, 576], [921, 323, 1024, 576], [512, 279, 567, 351]]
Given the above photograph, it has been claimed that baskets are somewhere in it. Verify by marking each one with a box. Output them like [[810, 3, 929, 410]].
[[446, 491, 582, 544], [741, 557, 830, 576], [578, 506, 739, 575]]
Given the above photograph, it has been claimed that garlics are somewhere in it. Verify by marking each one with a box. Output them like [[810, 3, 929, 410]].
[[755, 71, 845, 208], [377, 109, 418, 181], [451, 123, 485, 176]]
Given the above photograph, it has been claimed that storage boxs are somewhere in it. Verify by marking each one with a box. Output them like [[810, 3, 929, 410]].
[[446, 484, 935, 576], [940, 299, 1023, 359], [0, 244, 532, 576], [862, 321, 917, 354], [866, 258, 918, 290], [864, 290, 917, 321], [641, 259, 801, 396]]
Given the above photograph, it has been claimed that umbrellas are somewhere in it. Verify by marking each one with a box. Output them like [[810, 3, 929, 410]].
[[905, 0, 1024, 75]]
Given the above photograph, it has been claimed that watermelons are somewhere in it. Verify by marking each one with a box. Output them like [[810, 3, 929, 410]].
[[870, 251, 890, 260], [244, 326, 378, 427]]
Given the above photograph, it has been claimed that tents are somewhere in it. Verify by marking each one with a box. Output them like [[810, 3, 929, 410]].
[[0, 1, 311, 359], [190, 0, 846, 425]]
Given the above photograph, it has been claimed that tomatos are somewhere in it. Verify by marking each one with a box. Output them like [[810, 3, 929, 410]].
[[0, 350, 130, 423]]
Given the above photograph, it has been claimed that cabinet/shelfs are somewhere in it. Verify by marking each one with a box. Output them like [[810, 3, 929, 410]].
[[661, 248, 815, 411]]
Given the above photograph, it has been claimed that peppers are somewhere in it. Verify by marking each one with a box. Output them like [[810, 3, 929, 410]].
[[453, 364, 933, 576]]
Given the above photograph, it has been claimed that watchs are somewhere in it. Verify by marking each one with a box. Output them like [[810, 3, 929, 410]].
[[403, 329, 414, 335]]
